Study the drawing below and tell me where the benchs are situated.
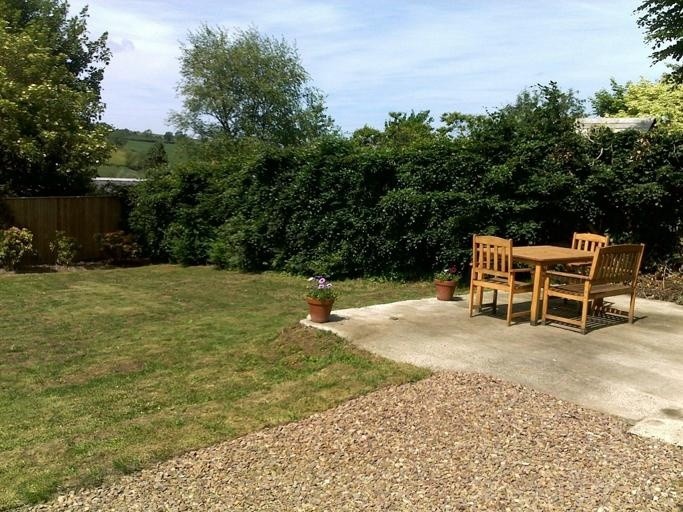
[542,242,644,334]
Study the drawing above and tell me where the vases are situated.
[306,296,333,322]
[434,279,457,301]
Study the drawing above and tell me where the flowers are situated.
[432,265,463,282]
[306,275,338,301]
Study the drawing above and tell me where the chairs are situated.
[469,232,608,326]
